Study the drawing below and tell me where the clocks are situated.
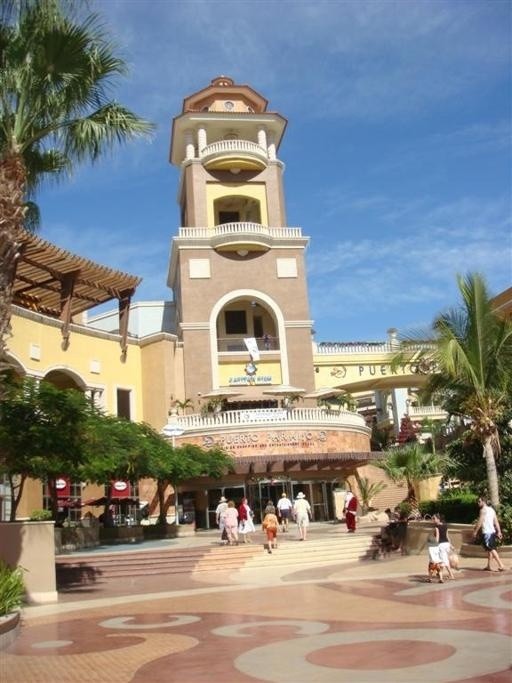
[245,362,256,375]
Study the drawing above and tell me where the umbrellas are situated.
[198,383,346,398]
[57,497,144,527]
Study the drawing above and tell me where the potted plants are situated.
[368,419,467,502]
[437,487,480,523]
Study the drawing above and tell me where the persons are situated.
[342,491,357,533]
[384,509,396,522]
[263,332,272,350]
[215,492,313,553]
[424,535,456,583]
[472,496,507,572]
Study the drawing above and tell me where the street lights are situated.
[161,407,187,524]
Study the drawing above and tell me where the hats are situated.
[296,492,306,498]
[219,496,228,503]
